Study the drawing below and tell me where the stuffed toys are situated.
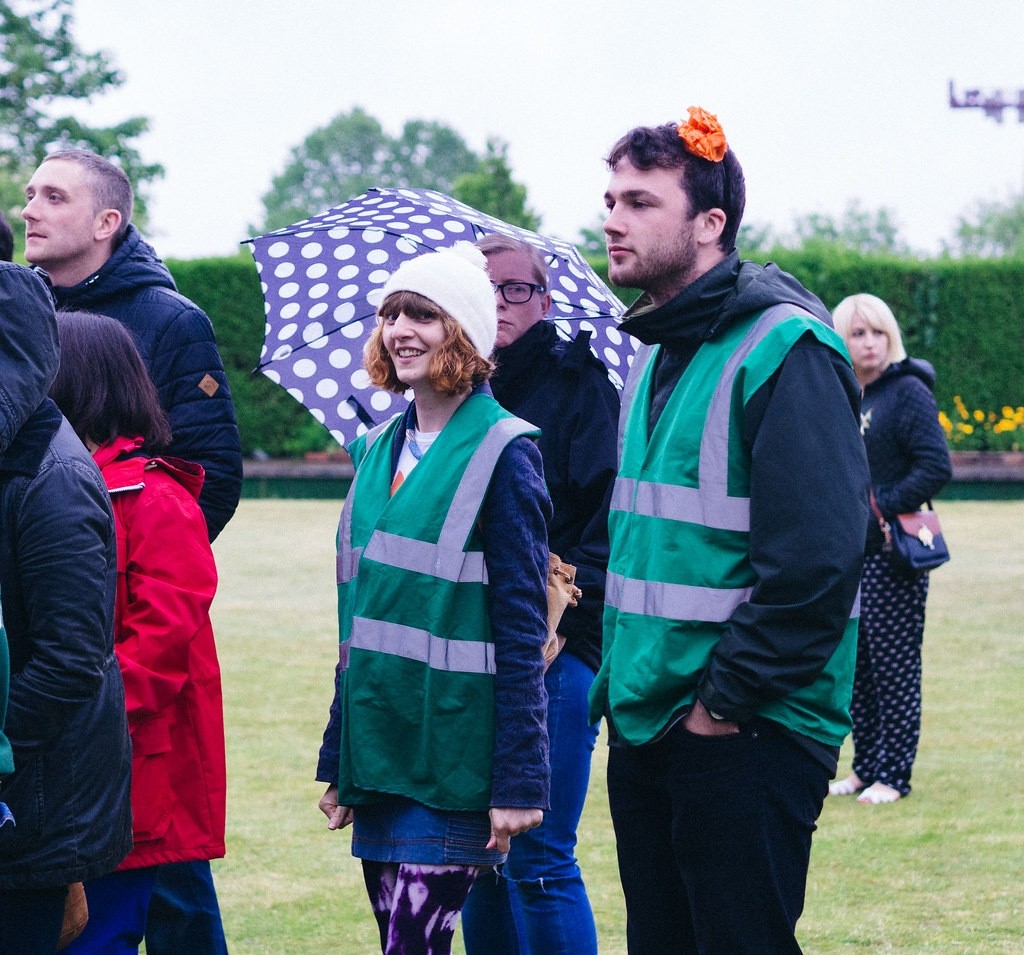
[540,553,582,670]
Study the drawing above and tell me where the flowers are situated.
[676,106,728,162]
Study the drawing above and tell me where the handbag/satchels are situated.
[869,488,950,571]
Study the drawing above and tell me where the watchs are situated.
[709,711,727,721]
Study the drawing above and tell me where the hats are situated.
[380,239,498,359]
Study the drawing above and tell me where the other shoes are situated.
[856,781,902,806]
[829,771,870,796]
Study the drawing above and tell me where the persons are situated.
[461,234,621,955]
[47,312,227,955]
[0,261,136,955]
[588,105,872,955]
[315,241,553,955]
[20,149,243,544]
[828,294,953,805]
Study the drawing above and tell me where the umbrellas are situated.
[240,186,643,454]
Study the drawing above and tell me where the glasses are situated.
[496,281,545,305]
